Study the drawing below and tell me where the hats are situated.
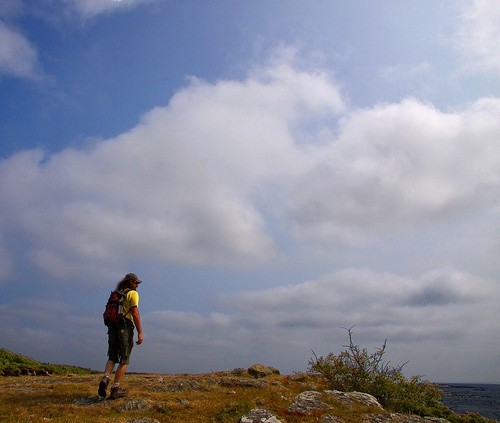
[125,273,142,284]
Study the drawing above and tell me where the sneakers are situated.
[98,377,108,398]
[111,386,128,399]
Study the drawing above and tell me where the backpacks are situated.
[103,290,123,328]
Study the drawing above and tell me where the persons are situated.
[98,273,143,399]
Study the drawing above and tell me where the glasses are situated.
[133,282,138,285]
